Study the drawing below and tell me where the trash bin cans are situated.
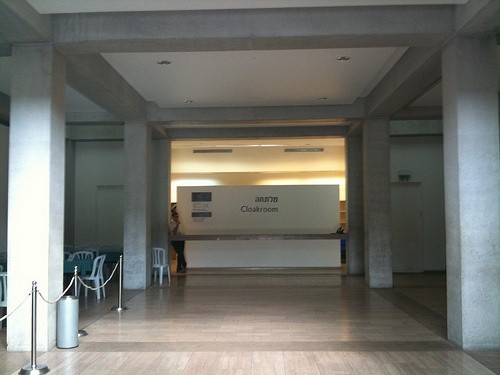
[55,294,80,349]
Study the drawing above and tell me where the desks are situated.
[97,249,124,280]
[63,259,99,297]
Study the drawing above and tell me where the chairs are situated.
[64,242,122,261]
[0,252,8,332]
[151,247,170,286]
[75,254,107,301]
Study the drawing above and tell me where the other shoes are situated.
[176,268,186,273]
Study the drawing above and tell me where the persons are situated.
[168,212,191,273]
